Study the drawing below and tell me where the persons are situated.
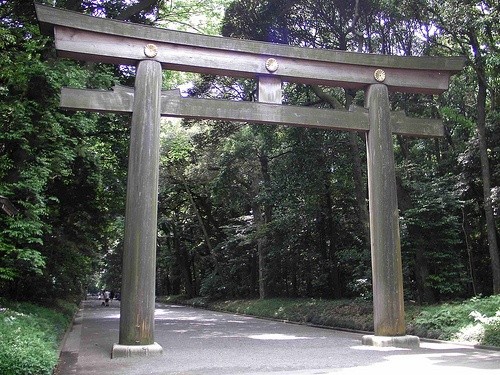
[97,289,120,306]
